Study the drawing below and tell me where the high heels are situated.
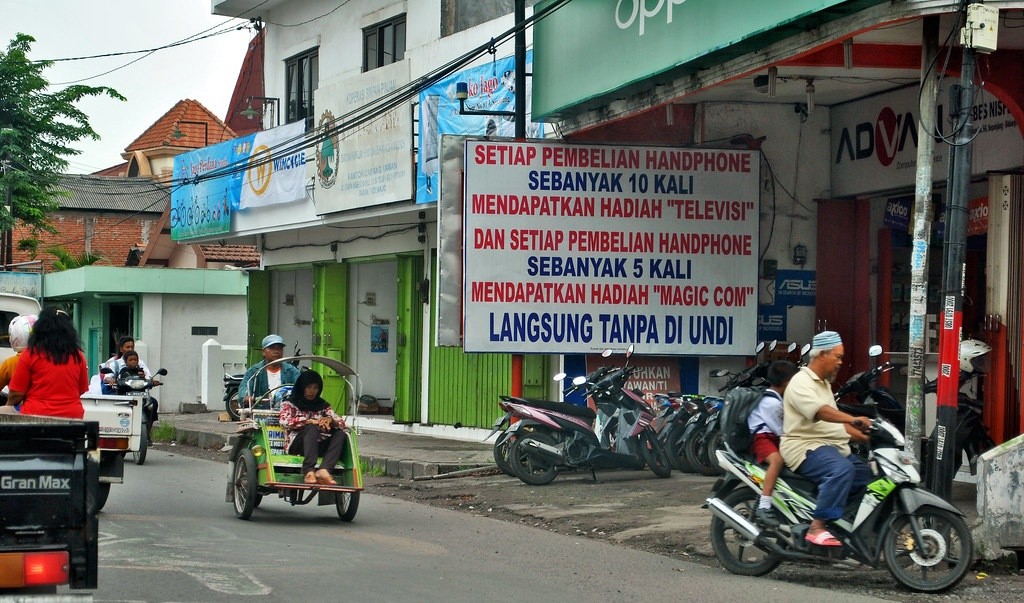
[317,476,338,485]
[304,475,316,483]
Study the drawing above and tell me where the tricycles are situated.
[221,356,363,520]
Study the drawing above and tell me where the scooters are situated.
[222,339,306,421]
[484,343,996,488]
[100,366,169,465]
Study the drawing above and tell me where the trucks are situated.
[0,293,144,512]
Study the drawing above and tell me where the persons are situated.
[109,350,139,386]
[746,360,796,526]
[0,313,40,392]
[279,370,345,486]
[238,335,300,410]
[779,331,873,546]
[100,356,118,395]
[103,337,160,428]
[6,304,89,420]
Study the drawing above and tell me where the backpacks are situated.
[720,387,781,454]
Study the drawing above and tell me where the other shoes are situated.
[148,436,153,446]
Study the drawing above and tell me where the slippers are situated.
[805,531,842,546]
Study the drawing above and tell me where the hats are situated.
[813,331,842,349]
[262,335,286,349]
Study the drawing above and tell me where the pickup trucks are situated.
[0,414,104,603]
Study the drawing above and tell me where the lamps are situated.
[170,120,209,147]
[239,95,280,126]
[455,81,516,119]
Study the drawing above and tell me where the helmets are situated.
[958,339,992,372]
[8,314,39,351]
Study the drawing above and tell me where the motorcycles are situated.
[696,399,975,593]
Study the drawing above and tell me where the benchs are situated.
[250,411,354,481]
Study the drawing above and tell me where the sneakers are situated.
[756,508,779,526]
